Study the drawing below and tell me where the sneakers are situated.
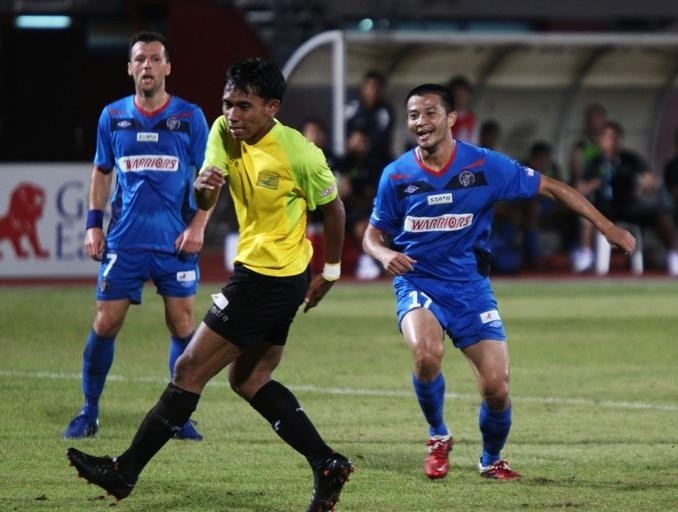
[478,456,525,481]
[63,410,100,441]
[423,432,454,478]
[306,451,356,511]
[172,420,205,441]
[66,446,136,503]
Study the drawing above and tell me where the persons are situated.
[362,82,636,479]
[65,55,355,512]
[65,29,209,440]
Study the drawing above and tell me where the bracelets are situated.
[85,210,105,229]
